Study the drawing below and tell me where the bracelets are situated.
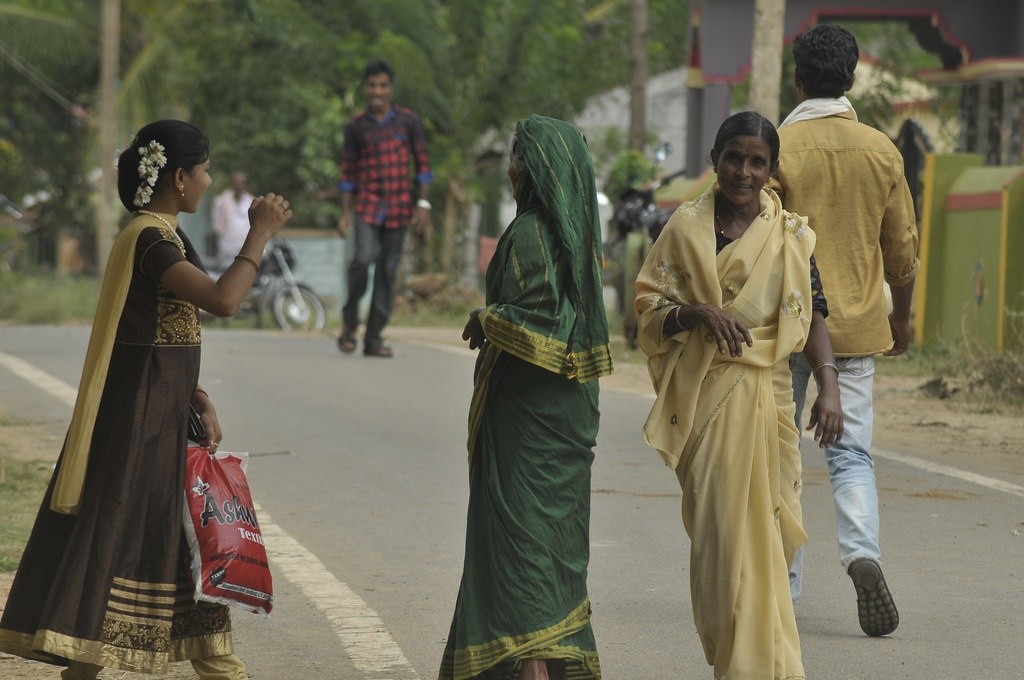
[813,362,840,386]
[196,384,209,397]
[235,254,259,274]
[416,198,432,210]
[675,306,687,332]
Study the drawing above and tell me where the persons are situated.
[0,120,295,680]
[210,173,270,273]
[337,61,432,357]
[605,204,653,348]
[633,112,844,680]
[438,114,614,680]
[763,26,921,635]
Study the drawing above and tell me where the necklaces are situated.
[132,210,186,254]
[715,201,726,246]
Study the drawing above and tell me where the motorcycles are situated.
[591,142,692,247]
[194,227,325,337]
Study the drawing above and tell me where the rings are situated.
[212,442,218,447]
[281,203,286,211]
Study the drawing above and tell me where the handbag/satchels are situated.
[183,439,273,617]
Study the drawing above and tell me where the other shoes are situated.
[848,558,899,636]
[364,340,392,356]
[339,320,356,352]
